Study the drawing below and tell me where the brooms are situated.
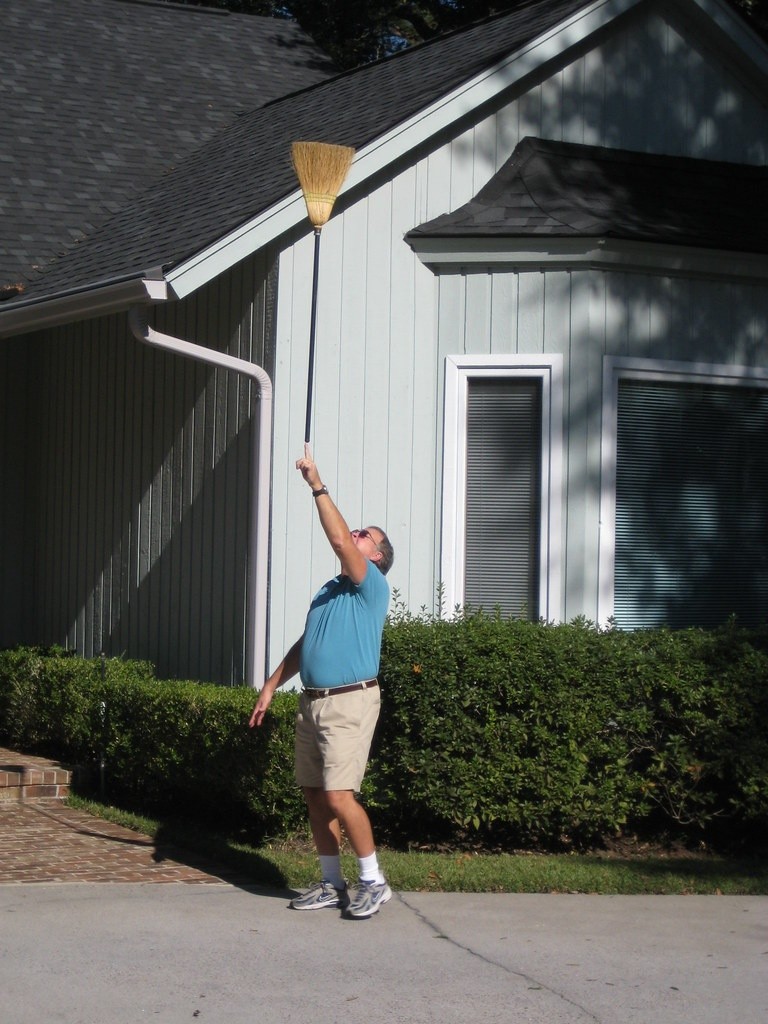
[288,139,357,442]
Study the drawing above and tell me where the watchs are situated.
[313,484,329,497]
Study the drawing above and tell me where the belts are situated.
[307,679,377,698]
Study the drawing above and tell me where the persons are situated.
[246,442,394,920]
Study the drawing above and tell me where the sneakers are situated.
[290,878,351,910]
[345,876,392,918]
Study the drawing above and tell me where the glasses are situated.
[350,529,382,552]
